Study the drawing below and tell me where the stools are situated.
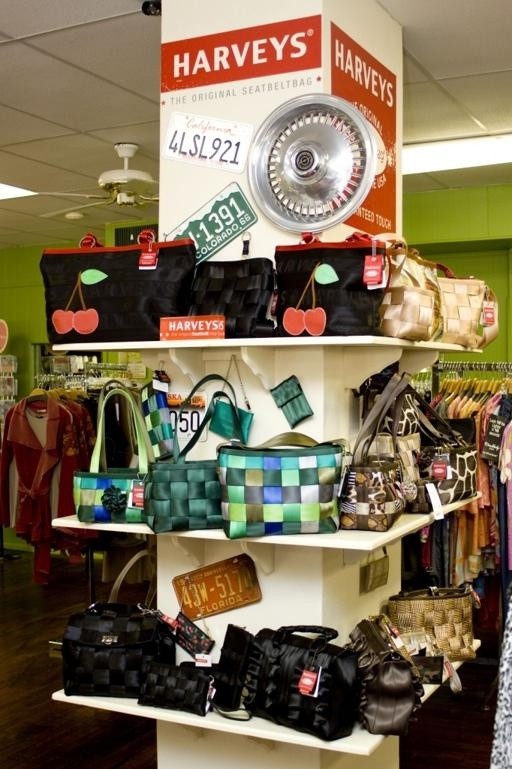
[48,523,136,651]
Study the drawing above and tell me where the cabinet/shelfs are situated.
[51,335,484,769]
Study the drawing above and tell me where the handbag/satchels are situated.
[374,432,422,484]
[39,229,196,344]
[340,453,407,532]
[208,399,253,444]
[187,257,275,338]
[71,464,156,525]
[365,556,390,593]
[273,231,391,337]
[360,385,420,435]
[376,240,445,343]
[436,263,500,351]
[210,623,267,721]
[217,434,354,540]
[344,614,425,736]
[170,575,215,662]
[144,458,246,535]
[389,583,482,661]
[137,661,217,717]
[270,375,313,430]
[419,419,477,447]
[253,626,362,742]
[138,370,176,461]
[410,656,444,684]
[405,442,482,515]
[61,602,175,696]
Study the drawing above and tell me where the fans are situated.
[38,142,159,218]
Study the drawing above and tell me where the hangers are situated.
[25,373,89,402]
[87,362,128,382]
[437,361,512,405]
